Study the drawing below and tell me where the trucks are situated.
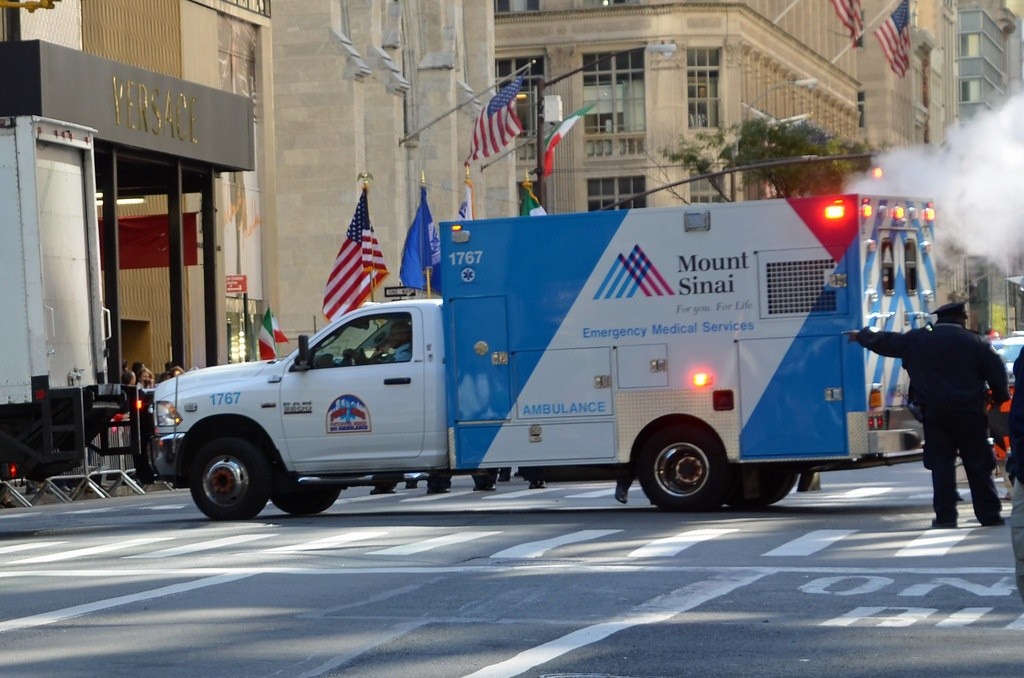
[1,116,143,511]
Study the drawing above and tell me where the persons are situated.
[615,477,638,505]
[841,299,1010,529]
[375,322,412,364]
[1006,344,1024,609]
[120,360,200,487]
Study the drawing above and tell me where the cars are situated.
[984,330,1024,415]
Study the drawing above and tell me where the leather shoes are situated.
[614,484,628,503]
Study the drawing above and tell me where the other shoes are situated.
[981,516,1005,526]
[932,518,959,528]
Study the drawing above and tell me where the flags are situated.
[457,179,476,221]
[520,186,548,216]
[833,0,866,48]
[399,186,442,296]
[323,186,390,322]
[543,103,600,178]
[465,67,524,167]
[873,0,910,78]
[259,306,288,360]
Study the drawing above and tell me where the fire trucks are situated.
[146,192,949,521]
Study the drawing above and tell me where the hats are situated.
[930,300,968,319]
